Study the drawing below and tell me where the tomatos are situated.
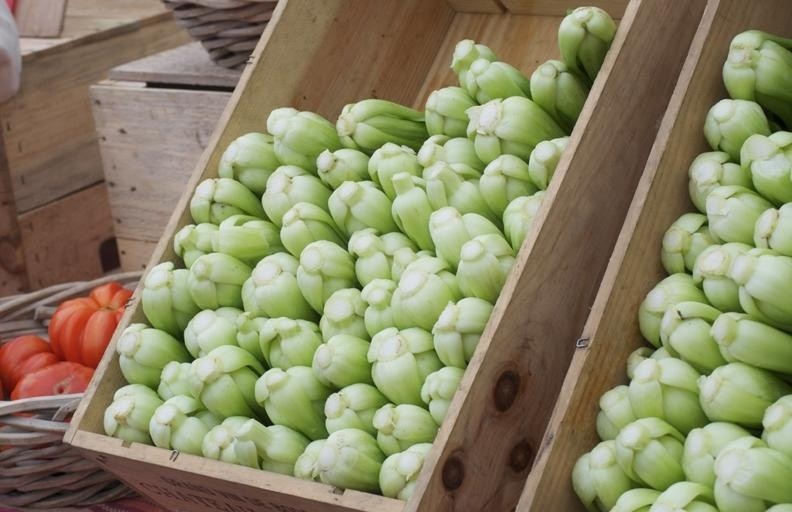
[0,280,139,453]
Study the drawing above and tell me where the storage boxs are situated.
[63,0,705,512]
[2,0,195,298]
[84,39,259,271]
[515,1,792,511]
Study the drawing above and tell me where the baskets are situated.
[2,271,142,512]
[160,0,277,72]
